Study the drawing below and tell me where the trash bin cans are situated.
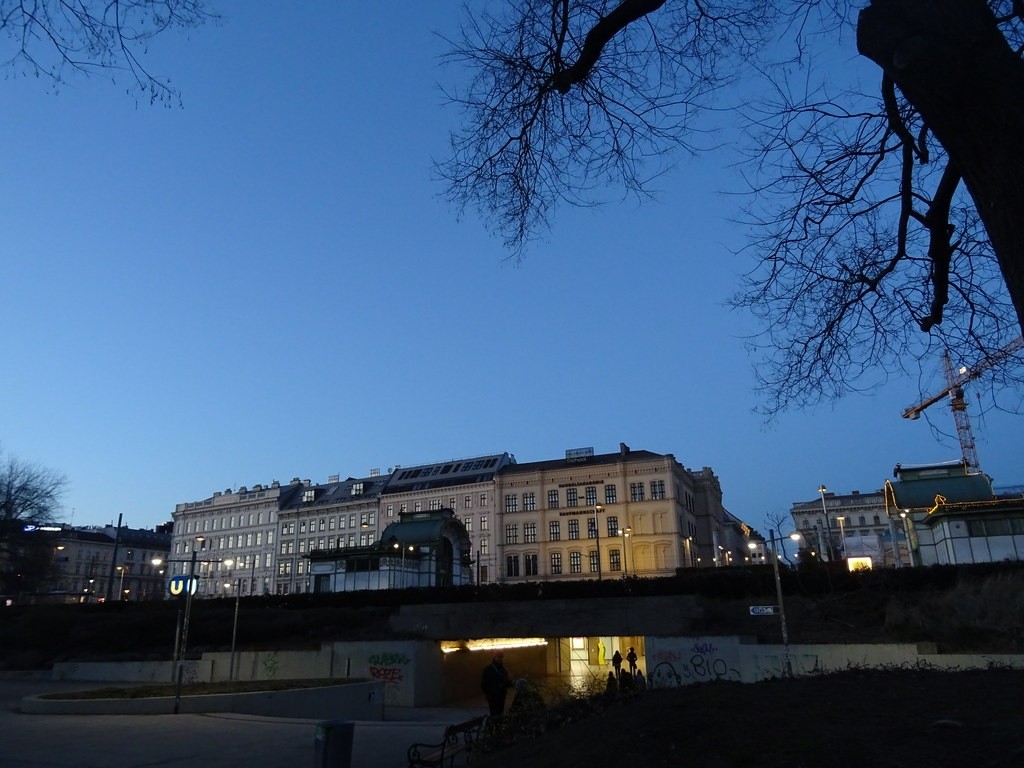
[314,721,357,768]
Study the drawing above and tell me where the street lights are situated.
[148,549,237,716]
[836,514,848,559]
[594,501,603,581]
[239,559,256,595]
[223,578,243,683]
[747,530,801,701]
[817,484,836,560]
[618,525,633,575]
[393,541,415,589]
[116,565,125,600]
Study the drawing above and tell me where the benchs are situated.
[407,714,488,768]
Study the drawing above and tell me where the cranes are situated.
[898,334,1024,474]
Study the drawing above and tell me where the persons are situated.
[509,678,544,735]
[480,650,517,735]
[603,669,646,695]
[626,647,637,679]
[612,651,623,676]
